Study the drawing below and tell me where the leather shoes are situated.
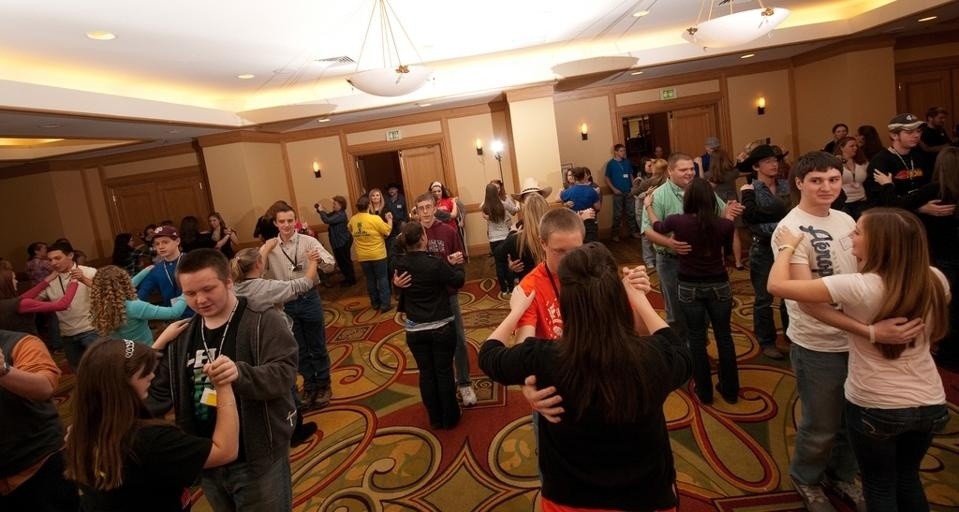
[693,385,714,404]
[430,405,463,430]
[734,261,745,270]
[290,422,317,448]
[761,346,784,358]
[715,383,738,405]
[372,303,394,312]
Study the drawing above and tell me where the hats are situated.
[511,178,553,203]
[738,144,789,173]
[705,137,720,149]
[888,113,928,132]
[151,226,179,238]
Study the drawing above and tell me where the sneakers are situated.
[459,385,478,408]
[612,235,620,242]
[339,277,357,288]
[646,263,655,269]
[632,231,641,238]
[790,477,866,512]
[311,384,332,409]
[299,388,317,409]
[502,287,510,296]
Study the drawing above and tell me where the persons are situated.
[641,152,747,404]
[389,193,477,431]
[604,137,745,269]
[346,181,470,313]
[729,105,958,509]
[315,196,357,288]
[477,207,692,509]
[1,200,334,511]
[480,166,601,300]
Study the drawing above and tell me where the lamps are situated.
[312,161,321,178]
[681,0,792,50]
[581,122,588,140]
[757,96,766,115]
[476,139,483,155]
[491,139,504,186]
[346,0,432,97]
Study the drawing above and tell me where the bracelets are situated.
[869,324,876,344]
